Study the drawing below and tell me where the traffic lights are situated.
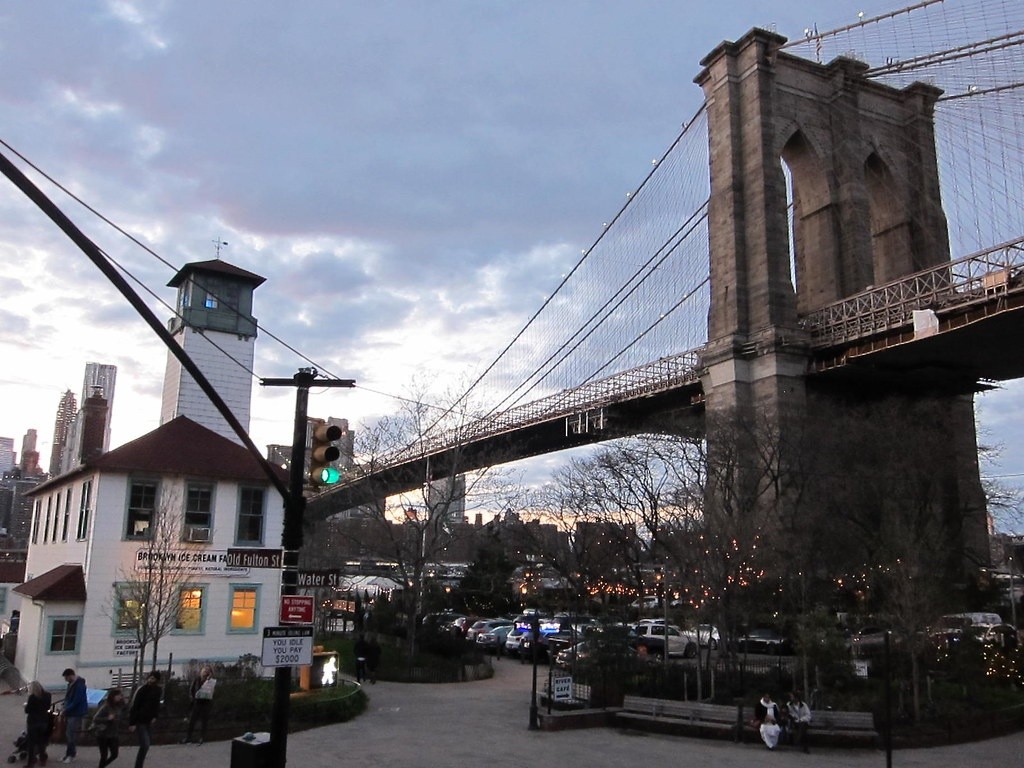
[309,422,342,487]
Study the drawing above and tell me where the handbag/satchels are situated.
[195,678,217,700]
[749,719,762,729]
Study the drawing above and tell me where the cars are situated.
[628,595,666,608]
[719,627,791,653]
[420,608,667,669]
[629,623,700,659]
[680,623,732,651]
[844,624,905,648]
[911,613,1019,655]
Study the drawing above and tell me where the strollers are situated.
[7,707,61,763]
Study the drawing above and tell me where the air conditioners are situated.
[186,526,211,543]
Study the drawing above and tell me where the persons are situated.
[786,692,812,754]
[129,672,162,768]
[755,693,782,751]
[354,633,367,683]
[22,681,51,768]
[367,634,380,684]
[93,690,128,768]
[182,667,215,744]
[56,669,88,763]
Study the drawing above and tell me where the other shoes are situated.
[803,748,810,754]
[177,736,192,745]
[57,753,79,764]
[197,738,203,746]
[768,747,777,751]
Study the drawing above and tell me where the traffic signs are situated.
[278,595,315,625]
[554,677,573,701]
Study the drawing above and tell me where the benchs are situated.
[110,670,172,688]
[616,694,739,741]
[533,681,591,707]
[740,706,878,748]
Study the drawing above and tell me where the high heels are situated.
[23,756,38,768]
[40,754,48,767]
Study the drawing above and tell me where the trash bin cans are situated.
[231,732,270,768]
